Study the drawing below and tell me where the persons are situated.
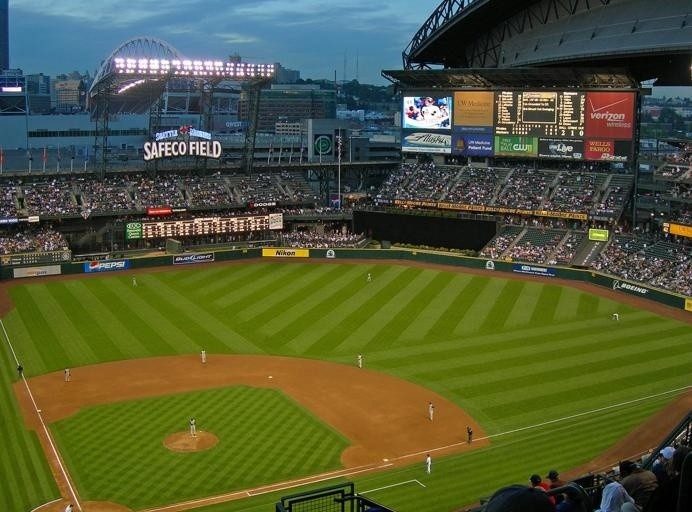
[65,504,73,512]
[407,97,449,126]
[480,422,691,512]
[189,417,196,438]
[358,354,362,368]
[425,453,432,473]
[428,402,434,421]
[17,365,23,375]
[200,348,206,363]
[466,426,472,444]
[64,367,70,382]
[612,313,618,320]
[2,144,692,297]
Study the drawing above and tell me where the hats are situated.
[660,447,676,459]
[471,484,554,512]
